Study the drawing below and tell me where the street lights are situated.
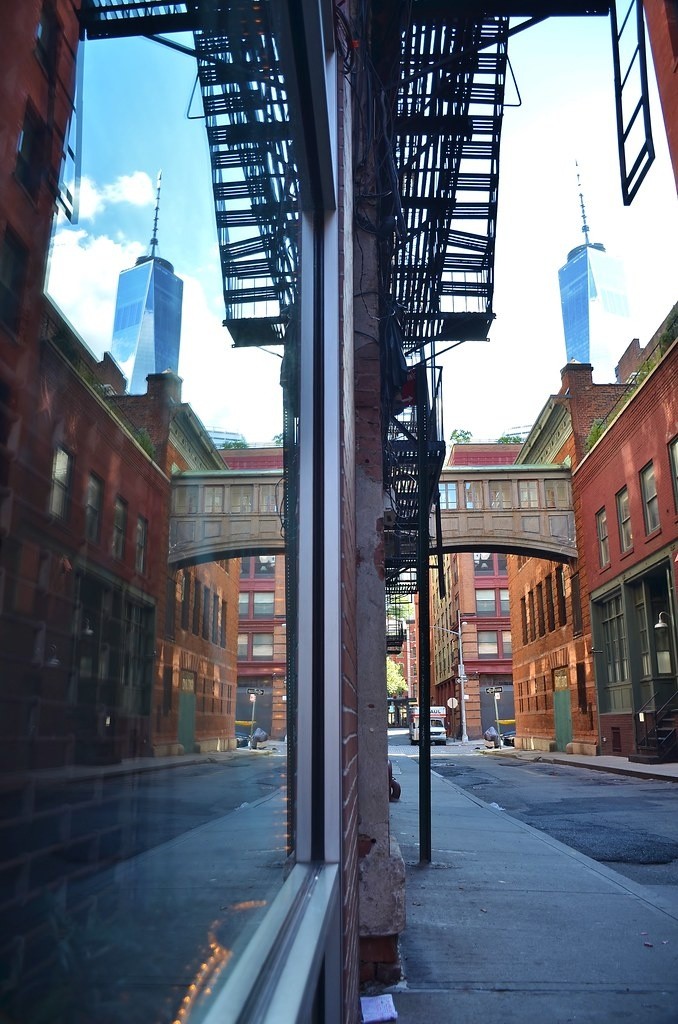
[416,608,470,744]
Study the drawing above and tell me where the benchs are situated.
[76,732,125,767]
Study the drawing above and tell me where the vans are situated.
[406,704,448,743]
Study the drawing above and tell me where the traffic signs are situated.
[486,687,503,693]
[246,688,264,695]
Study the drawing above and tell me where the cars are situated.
[500,730,516,747]
[235,731,250,749]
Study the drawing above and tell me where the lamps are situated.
[654,612,671,629]
[81,616,94,636]
[46,643,61,668]
